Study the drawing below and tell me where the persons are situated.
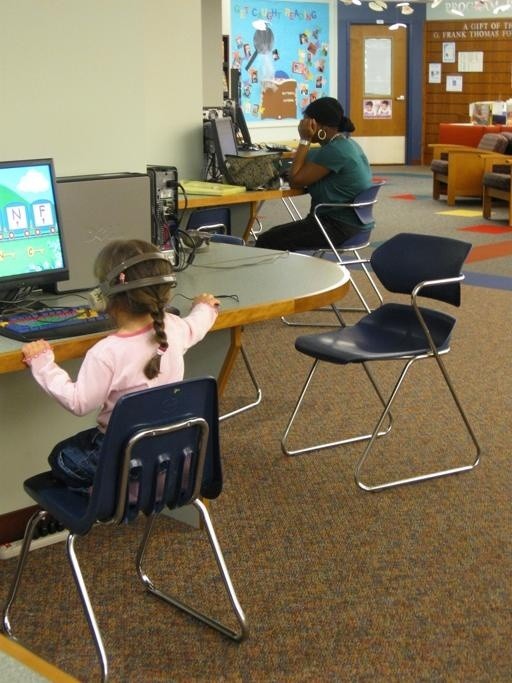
[364,101,376,117]
[254,96,374,251]
[472,103,495,123]
[19,239,221,510]
[378,100,391,117]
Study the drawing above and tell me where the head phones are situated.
[88,252,177,313]
[180,229,210,254]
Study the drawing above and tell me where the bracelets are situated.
[299,139,310,146]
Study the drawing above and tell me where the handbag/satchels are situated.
[223,150,284,191]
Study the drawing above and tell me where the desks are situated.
[0,241,353,425]
[172,180,310,244]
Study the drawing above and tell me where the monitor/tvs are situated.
[1,158,69,314]
[237,107,260,150]
[211,116,238,176]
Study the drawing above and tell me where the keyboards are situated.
[0,301,179,342]
[265,143,292,151]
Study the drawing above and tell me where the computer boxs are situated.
[203,106,225,181]
[147,162,177,251]
[42,173,150,296]
[225,99,239,135]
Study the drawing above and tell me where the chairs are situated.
[278,231,482,494]
[278,178,386,329]
[427,133,511,221]
[3,375,250,683]
